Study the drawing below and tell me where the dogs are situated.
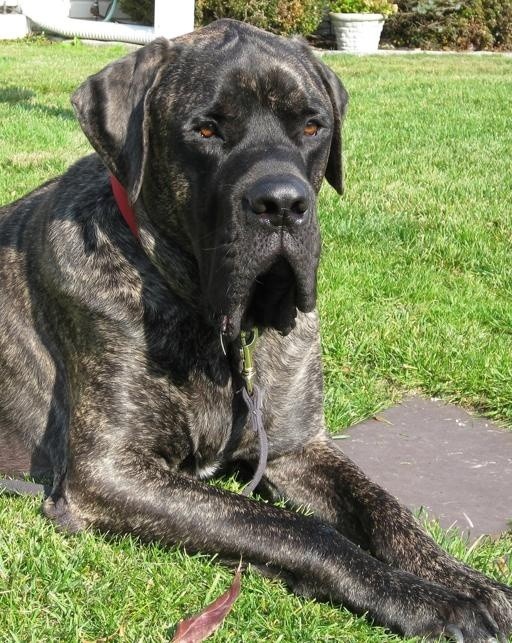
[0,18,512,643]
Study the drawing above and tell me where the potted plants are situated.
[328,0,397,55]
